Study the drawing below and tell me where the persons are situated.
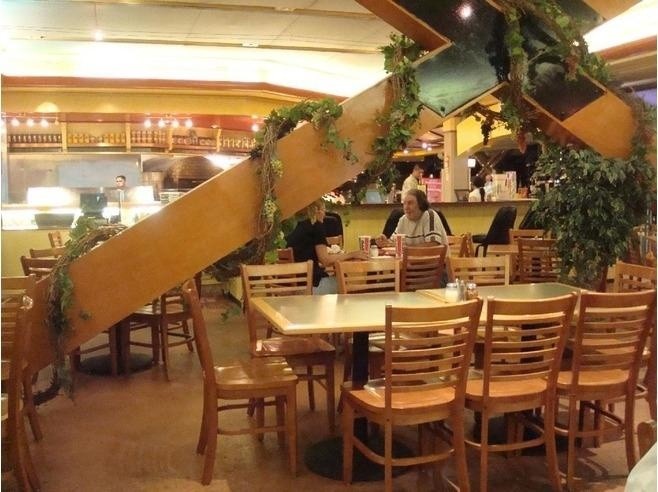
[284,197,369,294]
[110,176,130,202]
[375,189,449,288]
[468,176,487,203]
[400,164,424,203]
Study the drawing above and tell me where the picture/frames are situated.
[453,188,471,202]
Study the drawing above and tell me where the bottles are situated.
[132,130,215,146]
[445,278,465,303]
[370,244,379,257]
[466,282,479,300]
[67,132,126,145]
[7,135,62,142]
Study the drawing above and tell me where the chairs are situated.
[560,259,657,452]
[178,275,301,486]
[1,273,47,492]
[508,286,657,491]
[19,254,111,370]
[221,199,562,445]
[424,290,580,492]
[337,294,486,492]
[30,246,66,258]
[118,280,194,383]
[46,231,65,247]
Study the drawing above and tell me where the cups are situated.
[395,233,406,258]
[358,236,371,256]
[220,135,255,150]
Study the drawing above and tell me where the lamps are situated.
[2,112,59,128]
[142,114,195,131]
